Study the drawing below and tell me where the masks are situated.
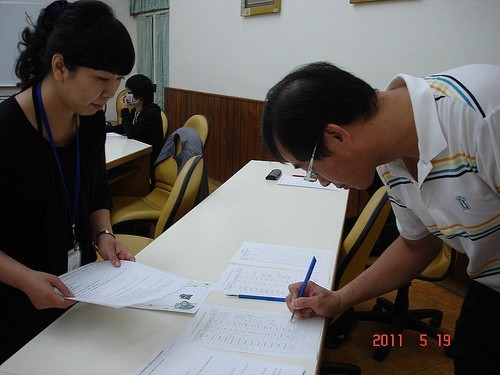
[125,94,142,106]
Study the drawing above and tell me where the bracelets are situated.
[92,230,117,251]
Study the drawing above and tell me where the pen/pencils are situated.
[292,174,306,178]
[291,255,318,320]
[225,294,286,303]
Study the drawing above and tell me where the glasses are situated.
[127,90,133,94]
[304,146,318,182]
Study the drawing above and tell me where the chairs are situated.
[321,186,458,375]
[96,89,209,263]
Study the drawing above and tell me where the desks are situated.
[105,132,153,169]
[0,160,349,375]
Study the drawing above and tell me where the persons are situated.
[104,74,163,197]
[260,62,500,375]
[0,0,135,365]
